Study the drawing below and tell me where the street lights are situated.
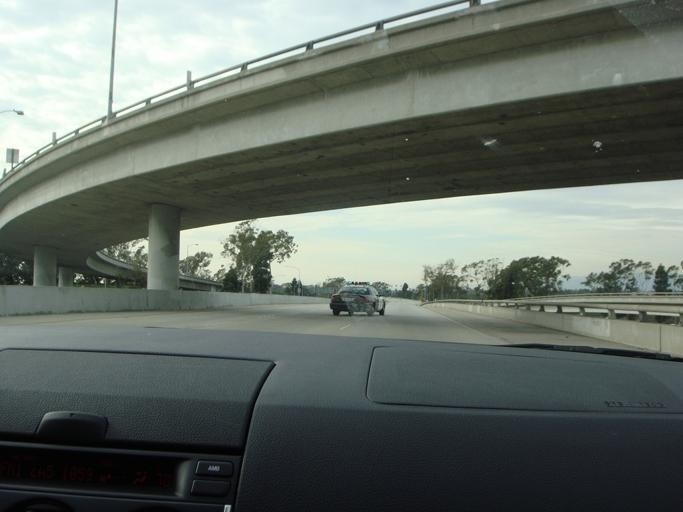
[0,108,23,116]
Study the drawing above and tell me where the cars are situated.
[330,283,385,317]
